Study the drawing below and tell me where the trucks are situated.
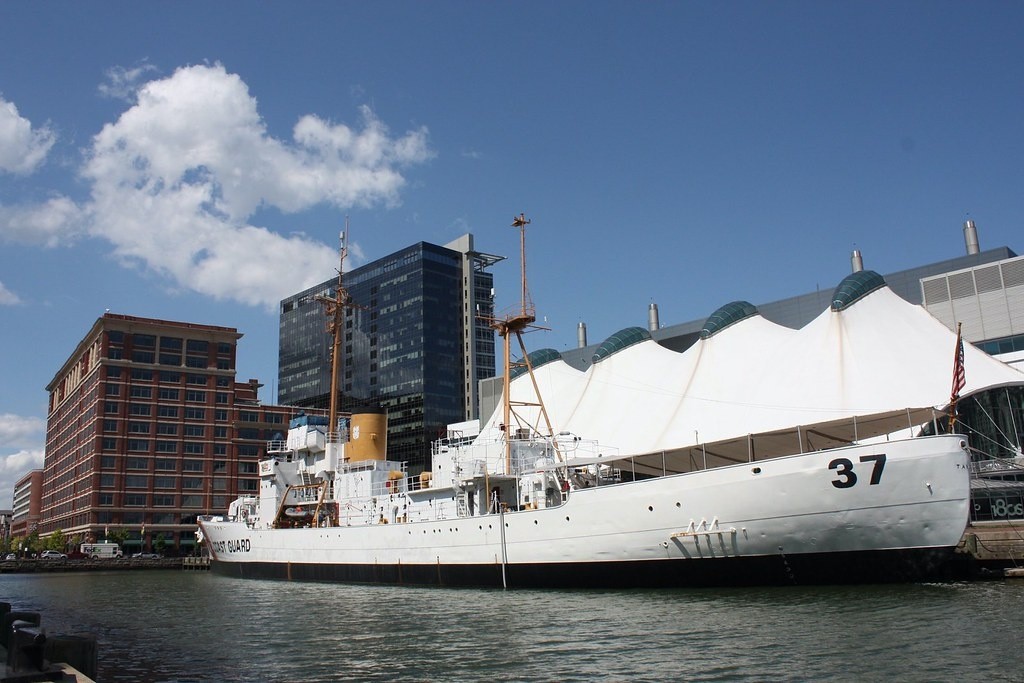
[80,543,123,559]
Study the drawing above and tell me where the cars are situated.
[132,552,162,559]
[6,553,16,560]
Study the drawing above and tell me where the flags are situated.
[951,329,966,401]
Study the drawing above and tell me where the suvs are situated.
[41,550,68,560]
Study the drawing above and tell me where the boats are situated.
[195,214,971,565]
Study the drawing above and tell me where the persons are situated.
[291,504,336,529]
